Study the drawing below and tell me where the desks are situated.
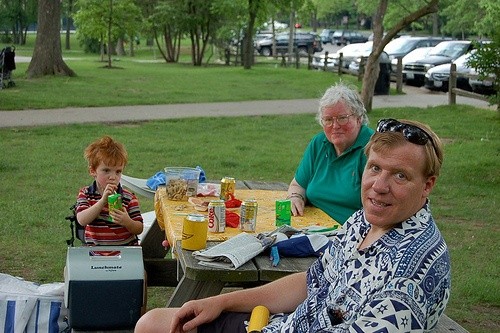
[139,181,342,308]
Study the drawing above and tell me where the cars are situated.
[315,37,500,91]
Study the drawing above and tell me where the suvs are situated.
[332,30,368,46]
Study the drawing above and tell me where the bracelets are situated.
[286,191,304,201]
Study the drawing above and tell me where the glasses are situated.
[376,118,439,157]
[320,113,354,126]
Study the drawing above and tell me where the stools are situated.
[432,312,470,333]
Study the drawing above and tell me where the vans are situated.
[257,31,322,56]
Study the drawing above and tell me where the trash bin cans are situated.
[375,51,393,94]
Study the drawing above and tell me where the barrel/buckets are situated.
[165,167,201,202]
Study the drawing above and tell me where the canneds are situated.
[220,176,235,200]
[240,200,258,231]
[208,199,225,233]
[186,213,206,221]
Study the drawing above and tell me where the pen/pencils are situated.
[174,213,205,217]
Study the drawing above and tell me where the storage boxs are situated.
[64,246,145,328]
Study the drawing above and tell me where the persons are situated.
[285,78,375,224]
[134,117,452,332]
[75,135,144,245]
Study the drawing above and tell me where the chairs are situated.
[66,205,140,246]
